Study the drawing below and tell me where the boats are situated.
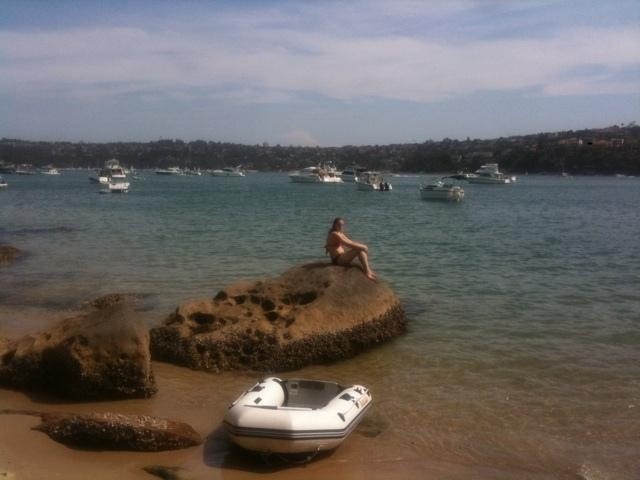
[0,162,61,191]
[419,176,464,202]
[439,161,516,184]
[289,157,391,191]
[153,164,248,176]
[224,377,372,457]
[98,157,135,192]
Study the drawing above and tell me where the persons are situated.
[324,217,379,283]
